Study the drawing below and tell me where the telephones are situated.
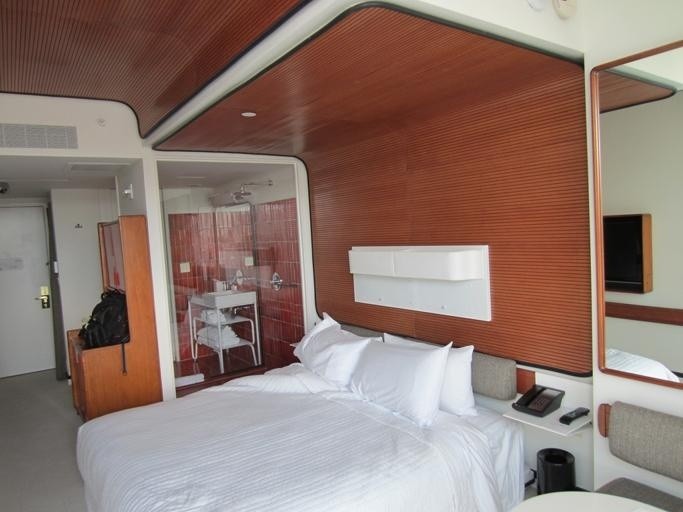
[512,384,565,417]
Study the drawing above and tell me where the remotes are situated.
[559,407,590,425]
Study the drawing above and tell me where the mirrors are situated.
[589,41,683,392]
[214,202,258,292]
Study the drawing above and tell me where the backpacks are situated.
[78,285,130,349]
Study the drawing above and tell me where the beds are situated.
[74,325,525,512]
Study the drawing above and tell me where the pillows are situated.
[291,311,479,426]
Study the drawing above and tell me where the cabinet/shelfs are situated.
[187,301,261,376]
[66,214,163,422]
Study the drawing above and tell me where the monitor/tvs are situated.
[602,214,652,293]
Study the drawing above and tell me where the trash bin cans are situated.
[537,448,575,494]
